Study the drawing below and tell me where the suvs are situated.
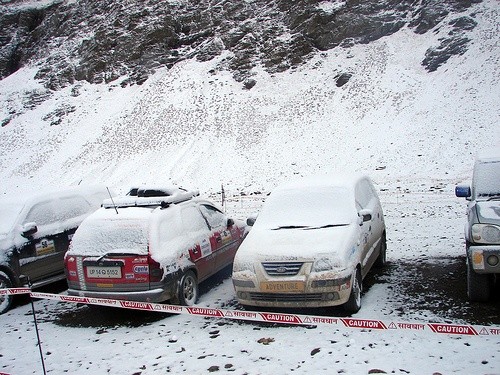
[455,148,500,301]
[63,184,250,307]
[0,186,104,315]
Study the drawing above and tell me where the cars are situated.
[231,172,388,314]
